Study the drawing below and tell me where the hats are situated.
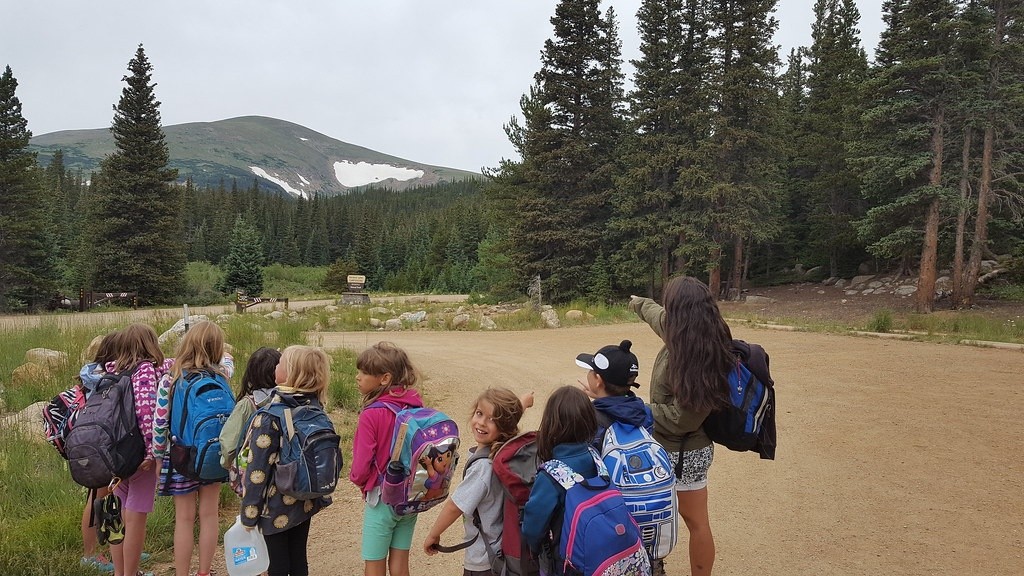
[575,340,640,389]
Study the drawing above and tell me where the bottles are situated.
[386,461,412,484]
[171,436,179,446]
[223,514,270,576]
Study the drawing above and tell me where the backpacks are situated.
[466,431,558,576]
[170,366,239,484]
[258,390,343,500]
[536,445,653,576]
[43,380,90,460]
[703,339,772,453]
[593,405,677,560]
[63,358,150,489]
[364,401,461,517]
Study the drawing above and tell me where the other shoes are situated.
[136,570,154,576]
[94,493,125,545]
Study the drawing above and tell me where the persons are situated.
[219,348,281,470]
[72,321,235,576]
[628,275,734,576]
[241,345,331,576]
[349,341,423,576]
[424,388,534,576]
[522,385,599,576]
[576,340,665,576]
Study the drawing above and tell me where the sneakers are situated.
[80,554,115,572]
[141,552,150,559]
